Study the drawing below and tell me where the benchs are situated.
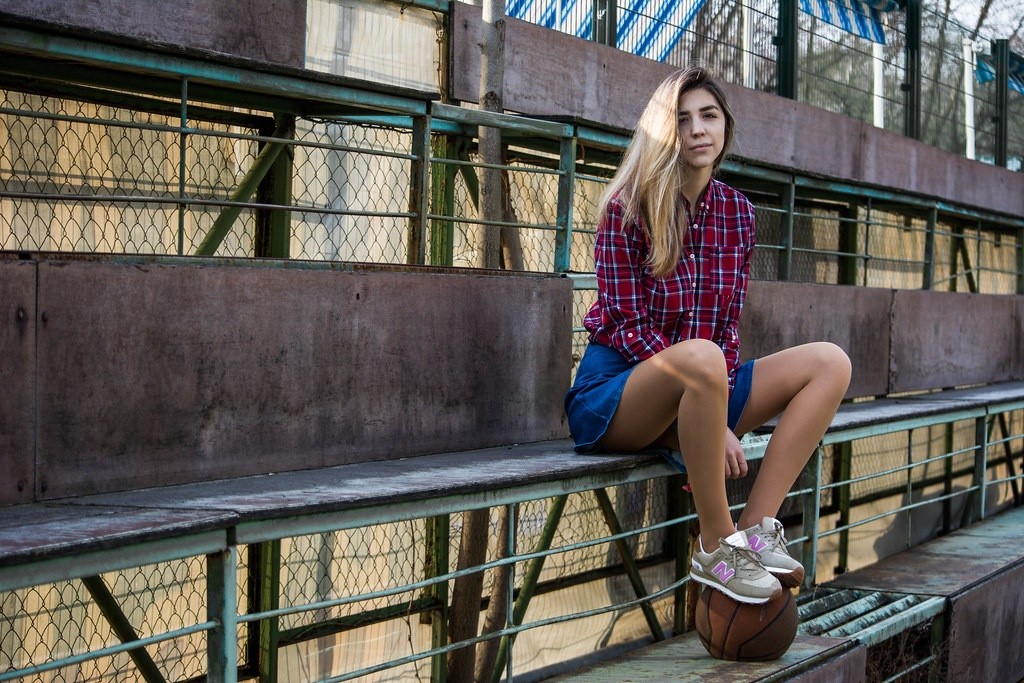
[0,250,1024,683]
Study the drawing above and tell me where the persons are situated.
[564,67,852,604]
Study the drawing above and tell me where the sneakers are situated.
[689,530,783,605]
[735,517,805,589]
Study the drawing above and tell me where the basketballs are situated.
[693,581,799,663]
